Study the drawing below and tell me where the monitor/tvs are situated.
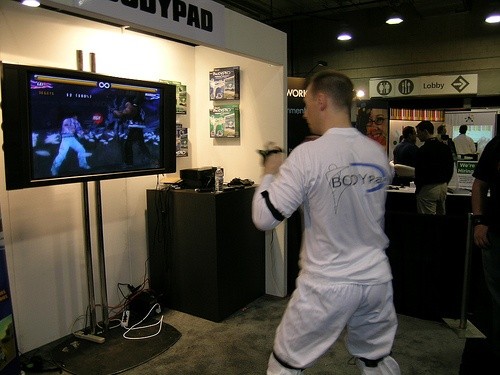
[0,63,177,191]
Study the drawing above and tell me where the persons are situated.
[393,126,420,189]
[252,70,399,375]
[436,124,448,144]
[471,133,500,289]
[452,124,476,155]
[414,121,455,215]
[367,108,390,150]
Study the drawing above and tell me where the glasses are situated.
[367,117,388,126]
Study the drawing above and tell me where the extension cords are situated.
[74,331,105,343]
[121,310,130,327]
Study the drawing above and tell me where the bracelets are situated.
[472,208,483,215]
